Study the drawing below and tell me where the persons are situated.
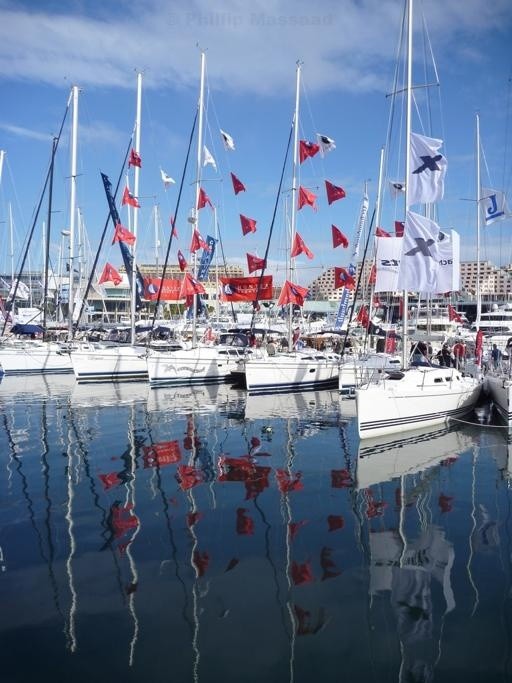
[490,344,503,369]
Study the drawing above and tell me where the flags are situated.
[490,189,505,227]
[94,132,483,367]
